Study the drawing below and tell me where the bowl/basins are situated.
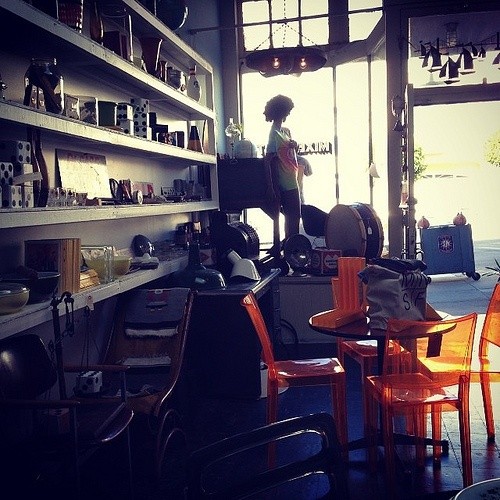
[0,283,30,315]
[0,272,61,303]
[84,259,130,281]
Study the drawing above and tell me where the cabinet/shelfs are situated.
[0,0,222,402]
[193,267,281,401]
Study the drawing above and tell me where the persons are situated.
[264,95,301,237]
[297,155,312,202]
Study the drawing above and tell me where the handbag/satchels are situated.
[357,265,428,332]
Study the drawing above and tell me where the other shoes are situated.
[285,271,307,277]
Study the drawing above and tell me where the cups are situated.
[113,255,131,275]
[48,185,88,206]
[236,139,252,158]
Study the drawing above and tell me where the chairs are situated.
[0,257,500,500]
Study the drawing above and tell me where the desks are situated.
[309,309,457,450]
[217,157,283,247]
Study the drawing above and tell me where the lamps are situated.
[445,22,458,48]
[245,0,329,76]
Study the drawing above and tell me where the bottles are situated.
[187,124,202,152]
[225,117,239,159]
[27,0,187,95]
[186,60,200,102]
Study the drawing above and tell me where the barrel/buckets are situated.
[98,101,117,127]
